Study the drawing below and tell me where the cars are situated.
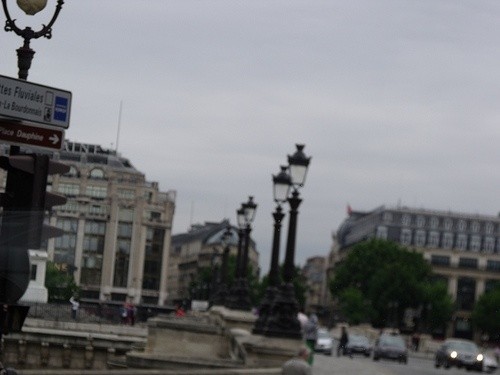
[373,335,409,363]
[433,339,483,372]
[313,331,334,356]
[343,334,373,357]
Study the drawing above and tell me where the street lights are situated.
[262,143,313,338]
[228,195,258,311]
[216,228,232,305]
[250,165,293,335]
[224,204,246,308]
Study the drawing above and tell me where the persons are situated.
[303,309,318,365]
[412,329,421,352]
[481,333,500,361]
[119,303,139,326]
[70,296,80,319]
[176,304,185,318]
[336,326,349,357]
